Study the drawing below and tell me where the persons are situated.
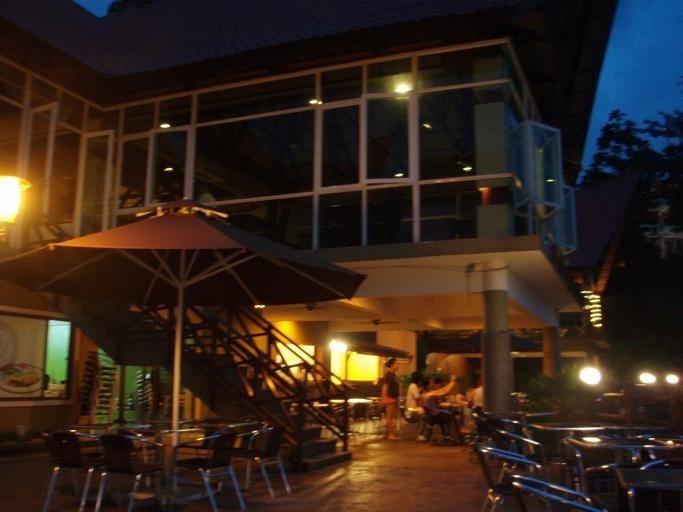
[380,356,401,440]
[403,371,485,443]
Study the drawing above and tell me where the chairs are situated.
[40,416,292,510]
[474,395,683,511]
[291,395,485,448]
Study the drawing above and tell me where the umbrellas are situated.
[0,195,367,490]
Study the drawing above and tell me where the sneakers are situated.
[417,434,461,445]
[385,432,399,440]
